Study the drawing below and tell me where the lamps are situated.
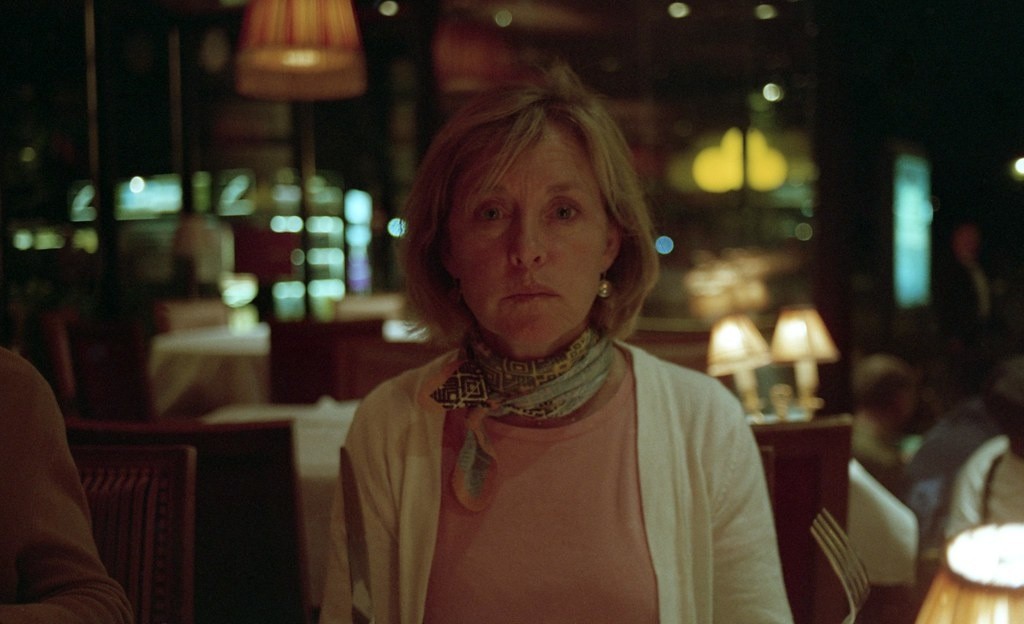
[706,304,841,422]
[235,0,368,103]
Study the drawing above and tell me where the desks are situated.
[145,322,270,415]
[205,401,358,607]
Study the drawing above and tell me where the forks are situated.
[811,508,872,624]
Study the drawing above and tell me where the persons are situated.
[936,222,996,355]
[318,65,793,624]
[849,353,917,489]
[0,346,135,624]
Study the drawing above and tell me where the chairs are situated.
[75,445,197,624]
[63,413,321,624]
